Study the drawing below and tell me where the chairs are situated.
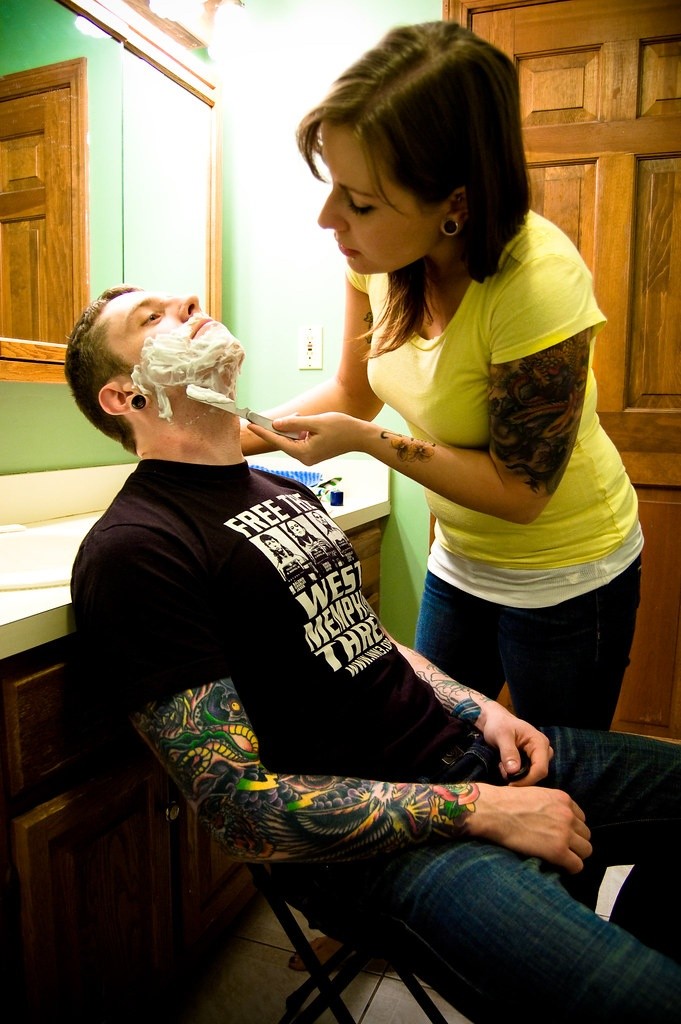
[245,860,446,1022]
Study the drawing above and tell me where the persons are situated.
[240,20,645,972]
[64,285,681,1024]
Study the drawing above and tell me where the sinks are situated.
[1,534,82,580]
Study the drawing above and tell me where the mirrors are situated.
[0,0,228,387]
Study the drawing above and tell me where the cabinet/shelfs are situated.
[0,631,181,1023]
[180,733,259,964]
[345,525,381,619]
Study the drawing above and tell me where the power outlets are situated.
[297,324,323,370]
